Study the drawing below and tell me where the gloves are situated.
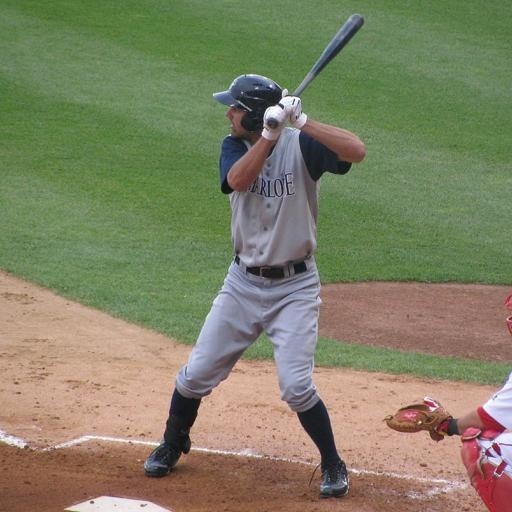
[260,107,286,142]
[278,96,308,131]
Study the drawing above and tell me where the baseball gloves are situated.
[385,397,454,439]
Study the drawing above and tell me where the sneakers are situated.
[316,458,347,498]
[143,441,181,478]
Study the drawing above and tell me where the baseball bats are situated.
[268,13,364,130]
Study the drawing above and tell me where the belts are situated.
[233,252,307,278]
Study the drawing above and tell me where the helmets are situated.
[209,72,286,114]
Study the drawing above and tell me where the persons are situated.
[383,287,512,512]
[144,74,367,497]
